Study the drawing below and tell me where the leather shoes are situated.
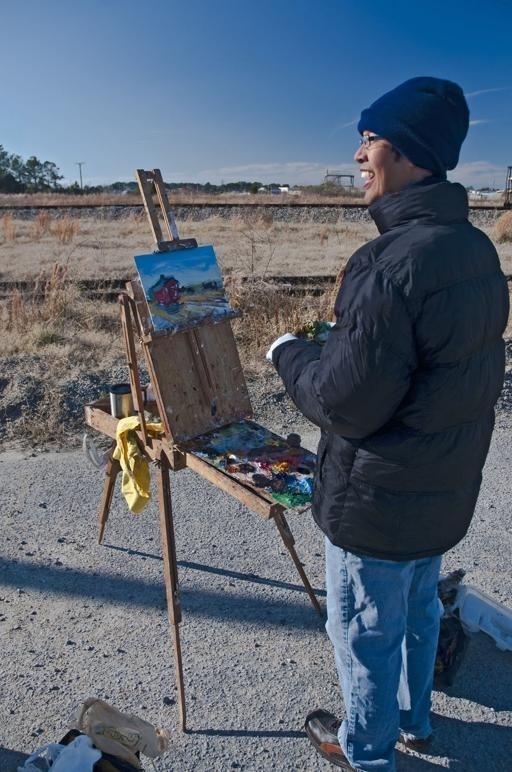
[305,707,357,772]
[396,733,433,753]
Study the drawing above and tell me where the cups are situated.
[109,382,148,419]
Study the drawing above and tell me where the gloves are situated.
[315,322,337,348]
[265,330,299,365]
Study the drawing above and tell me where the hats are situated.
[357,75,470,175]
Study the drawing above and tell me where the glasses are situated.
[358,134,389,149]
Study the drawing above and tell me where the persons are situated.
[266,76,510,772]
